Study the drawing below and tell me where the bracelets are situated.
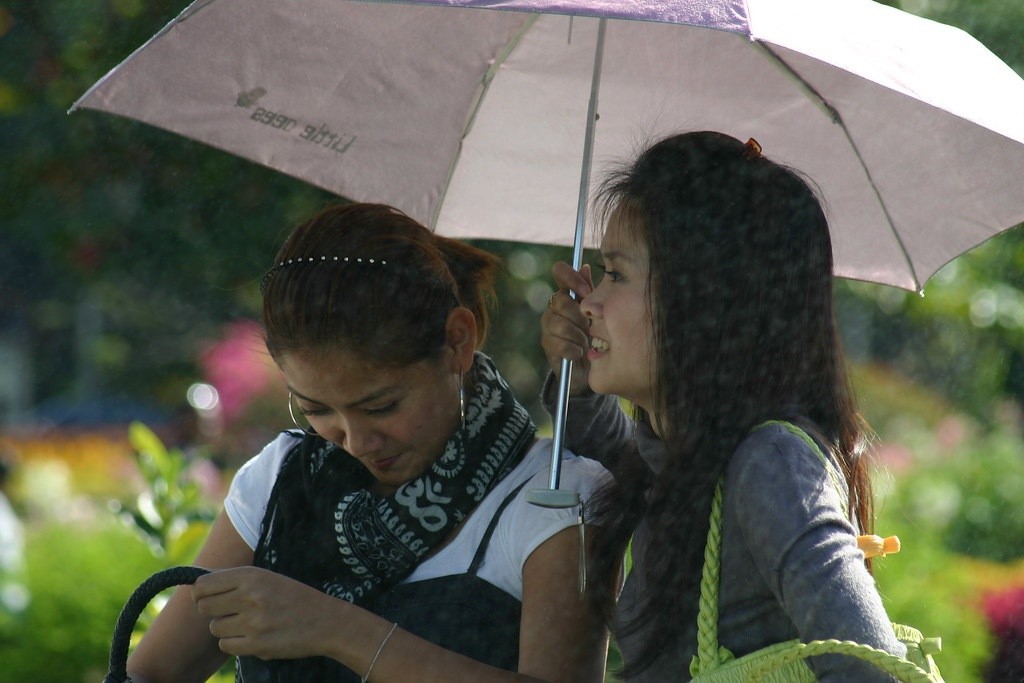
[362,623,398,683]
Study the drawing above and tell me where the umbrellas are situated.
[67,0,1024,508]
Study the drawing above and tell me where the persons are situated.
[539,131,908,683]
[127,203,626,683]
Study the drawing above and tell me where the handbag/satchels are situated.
[689,421,947,683]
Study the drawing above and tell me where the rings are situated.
[549,293,558,308]
